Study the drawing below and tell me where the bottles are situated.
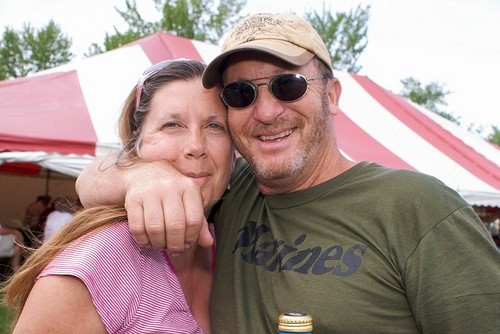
[278,312,313,334]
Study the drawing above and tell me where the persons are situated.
[75,13,500,334]
[0,193,81,272]
[0,57,238,334]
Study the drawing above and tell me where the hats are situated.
[201,12,333,90]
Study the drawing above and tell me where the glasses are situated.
[219,71,332,110]
[134,58,200,112]
